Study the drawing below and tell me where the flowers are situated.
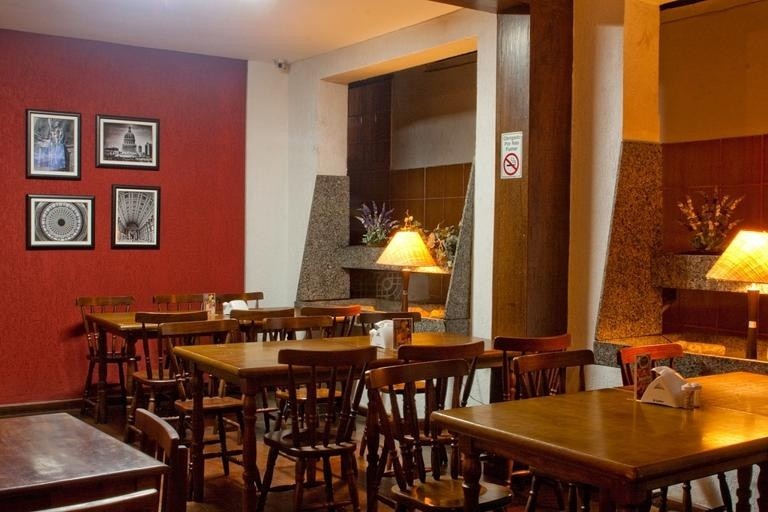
[350,199,398,247]
[673,181,743,254]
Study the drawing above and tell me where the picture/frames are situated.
[25,108,82,182]
[109,182,161,250]
[95,113,160,172]
[24,194,96,251]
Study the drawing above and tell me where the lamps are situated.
[374,228,438,309]
[704,228,767,360]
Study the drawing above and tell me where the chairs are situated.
[395,340,487,499]
[616,343,735,512]
[492,334,573,401]
[506,348,596,511]
[364,352,516,511]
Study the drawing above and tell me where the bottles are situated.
[223,302,231,314]
[680,382,702,409]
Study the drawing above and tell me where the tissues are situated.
[223,298,249,314]
[640,365,687,407]
[371,319,393,347]
